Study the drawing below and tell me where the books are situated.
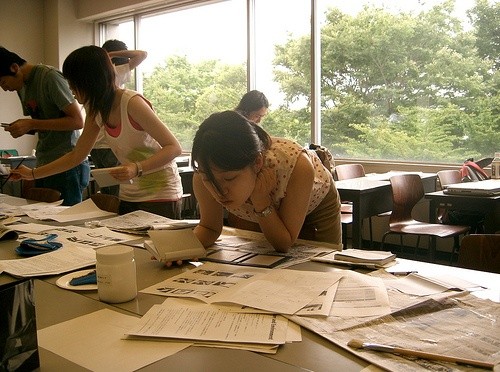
[334,249,396,266]
[91,166,133,188]
[143,229,206,264]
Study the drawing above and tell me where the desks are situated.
[0,156,36,197]
[424,189,500,264]
[177,166,198,219]
[332,171,438,263]
[0,194,500,372]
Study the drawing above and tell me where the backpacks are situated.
[458,158,496,183]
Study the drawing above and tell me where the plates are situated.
[56,269,99,290]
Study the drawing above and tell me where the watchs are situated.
[253,202,274,217]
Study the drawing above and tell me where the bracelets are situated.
[31,167,37,180]
[136,162,143,177]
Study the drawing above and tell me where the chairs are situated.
[335,163,365,180]
[381,175,468,265]
[460,234,500,273]
[20,188,61,203]
[0,149,22,195]
[416,170,463,262]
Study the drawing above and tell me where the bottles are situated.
[96,246,138,303]
[491,152,500,179]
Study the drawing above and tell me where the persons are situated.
[9,45,182,219]
[151,109,343,268]
[81,39,147,212]
[227,90,269,233]
[0,45,89,206]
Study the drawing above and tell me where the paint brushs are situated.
[346,338,495,369]
